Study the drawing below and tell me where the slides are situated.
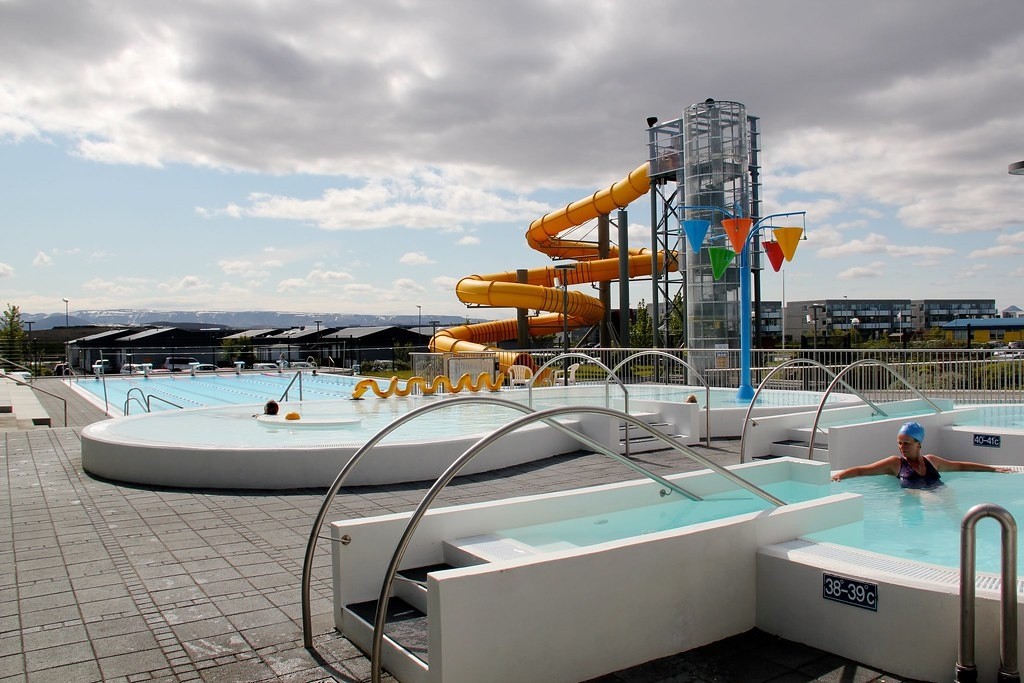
[428,154,681,383]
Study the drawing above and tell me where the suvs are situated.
[53,363,71,376]
[95,359,113,374]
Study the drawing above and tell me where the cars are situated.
[985,339,1024,360]
[190,364,221,370]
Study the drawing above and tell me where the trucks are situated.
[120,364,170,373]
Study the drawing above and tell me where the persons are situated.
[311,369,321,376]
[830,417,1019,530]
[680,392,701,406]
[216,400,279,420]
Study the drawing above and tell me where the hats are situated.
[899,421,925,442]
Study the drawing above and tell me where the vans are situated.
[165,357,201,371]
